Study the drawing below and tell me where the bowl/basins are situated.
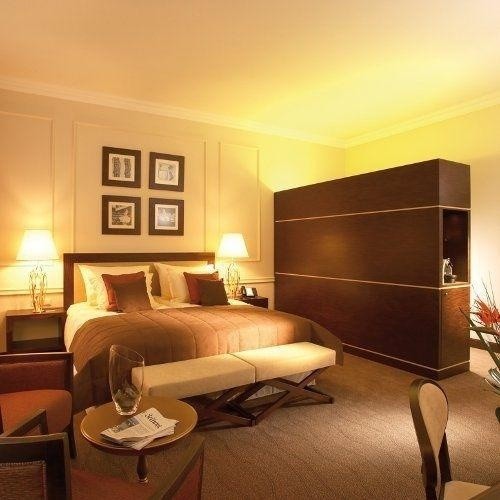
[445,275,457,283]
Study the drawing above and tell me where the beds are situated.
[63,252,344,428]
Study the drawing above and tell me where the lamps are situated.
[216,233,249,293]
[16,229,59,312]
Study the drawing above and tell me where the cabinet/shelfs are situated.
[274,158,471,381]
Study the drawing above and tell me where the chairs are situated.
[1,408,71,500]
[1,352,78,460]
[410,377,492,499]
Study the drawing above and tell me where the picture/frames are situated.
[102,194,141,235]
[149,151,185,193]
[101,146,142,188]
[149,197,184,235]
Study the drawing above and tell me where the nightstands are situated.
[6,310,67,355]
[234,295,268,309]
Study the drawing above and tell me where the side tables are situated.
[80,395,198,485]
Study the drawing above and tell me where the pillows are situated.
[78,262,159,312]
[154,262,229,306]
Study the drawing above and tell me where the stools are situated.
[131,341,336,427]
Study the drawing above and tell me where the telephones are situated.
[241,286,258,298]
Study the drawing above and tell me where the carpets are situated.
[0,408,205,500]
